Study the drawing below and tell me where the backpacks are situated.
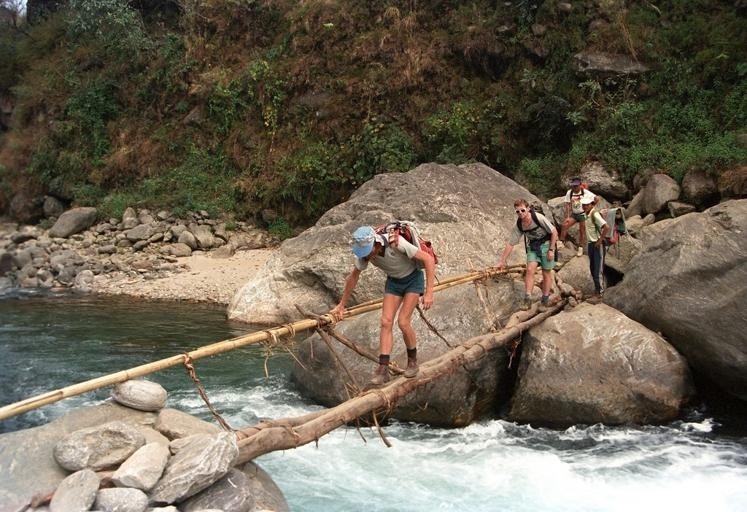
[373,219,439,265]
[591,206,626,245]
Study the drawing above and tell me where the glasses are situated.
[515,208,526,213]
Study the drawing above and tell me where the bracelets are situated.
[549,249,554,252]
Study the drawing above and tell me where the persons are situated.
[498,197,557,311]
[557,178,600,259]
[330,224,434,388]
[579,194,610,297]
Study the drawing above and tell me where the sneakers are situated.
[577,246,584,257]
[371,366,391,385]
[404,358,420,378]
[541,295,549,305]
[558,240,565,249]
[520,299,532,311]
[587,294,602,305]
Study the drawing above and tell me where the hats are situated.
[580,193,594,205]
[570,180,580,185]
[351,225,374,258]
